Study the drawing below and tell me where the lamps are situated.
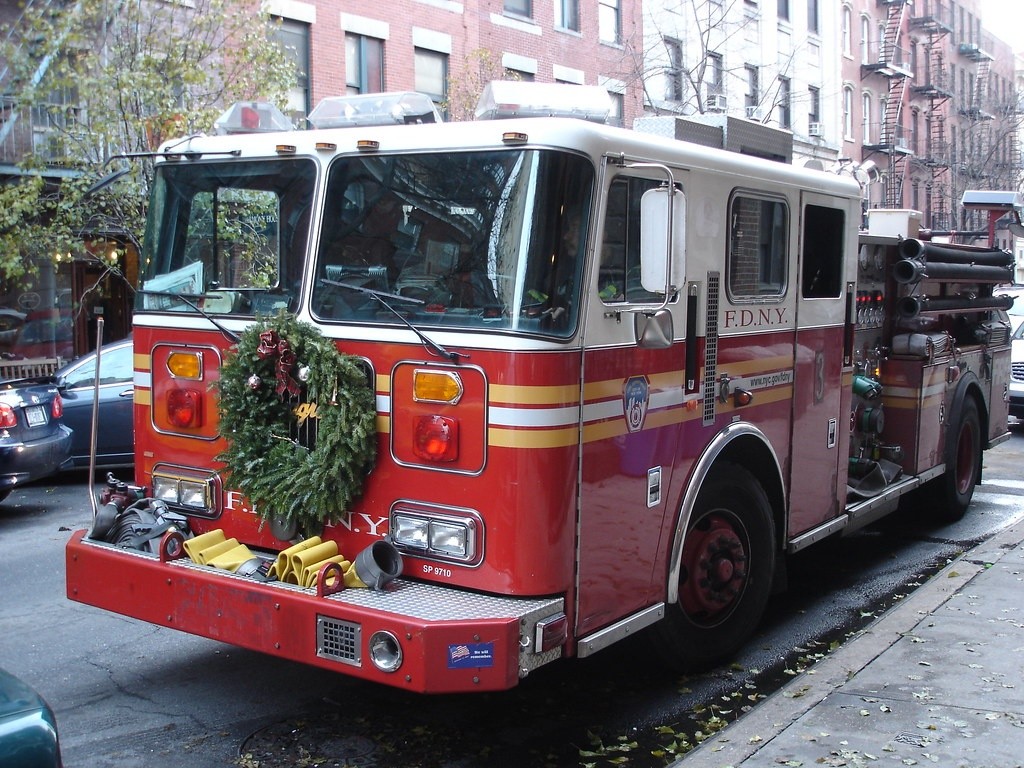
[825,158,881,234]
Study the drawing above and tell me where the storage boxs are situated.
[866,209,923,240]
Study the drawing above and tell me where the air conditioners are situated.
[746,105,763,120]
[809,122,825,136]
[707,93,727,111]
[895,137,907,148]
[897,62,911,70]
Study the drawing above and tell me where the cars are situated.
[44,337,133,478]
[0,371,75,507]
[991,287,1024,435]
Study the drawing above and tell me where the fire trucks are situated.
[60,77,1015,697]
